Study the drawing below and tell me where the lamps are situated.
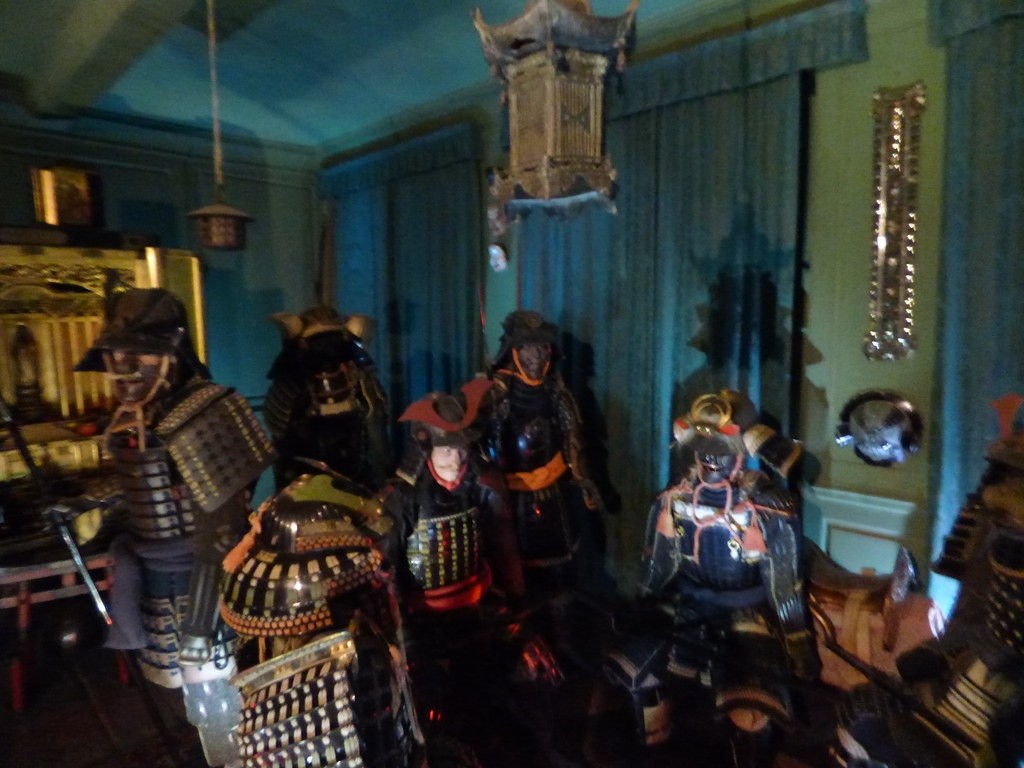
[185,1,258,252]
[471,1,637,215]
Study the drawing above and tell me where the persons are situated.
[20,287,280,768]
[264,305,391,491]
[897,425,1024,768]
[633,386,823,768]
[474,310,602,647]
[219,476,429,768]
[373,376,567,737]
